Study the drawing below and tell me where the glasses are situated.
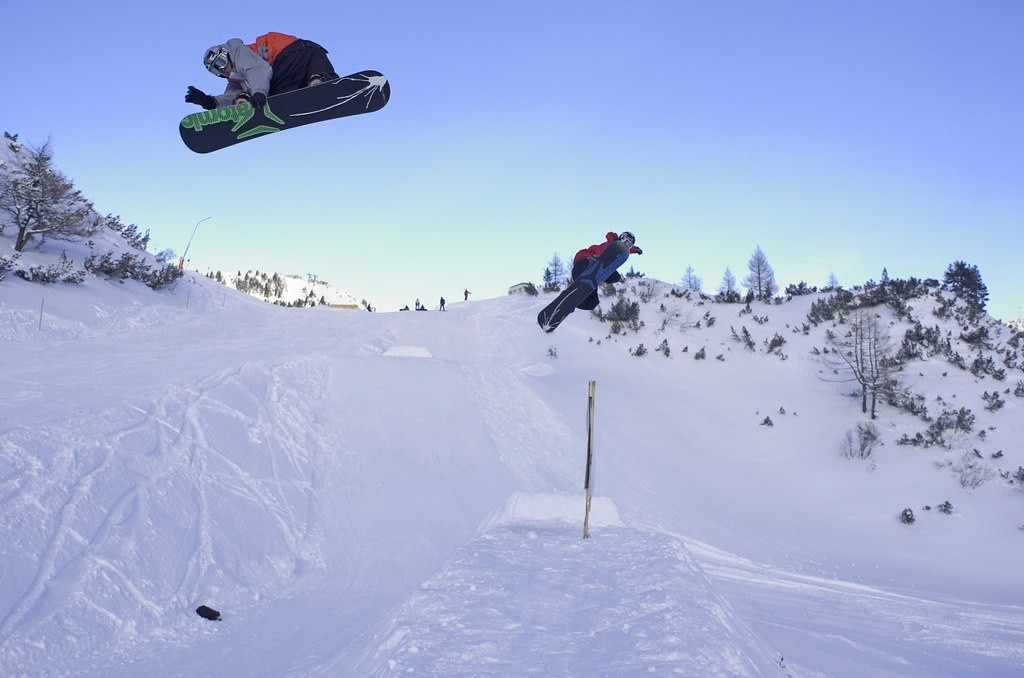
[209,48,228,76]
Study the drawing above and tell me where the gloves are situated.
[636,247,642,255]
[185,86,219,110]
[249,92,266,108]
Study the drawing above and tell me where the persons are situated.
[571,231,644,310]
[185,32,338,111]
[464,289,470,300]
[415,298,420,310]
[440,296,446,312]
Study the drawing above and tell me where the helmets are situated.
[204,45,233,78]
[619,232,635,249]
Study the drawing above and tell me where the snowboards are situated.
[537,240,630,333]
[178,69,392,156]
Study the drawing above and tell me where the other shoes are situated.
[235,91,250,105]
[309,73,326,87]
